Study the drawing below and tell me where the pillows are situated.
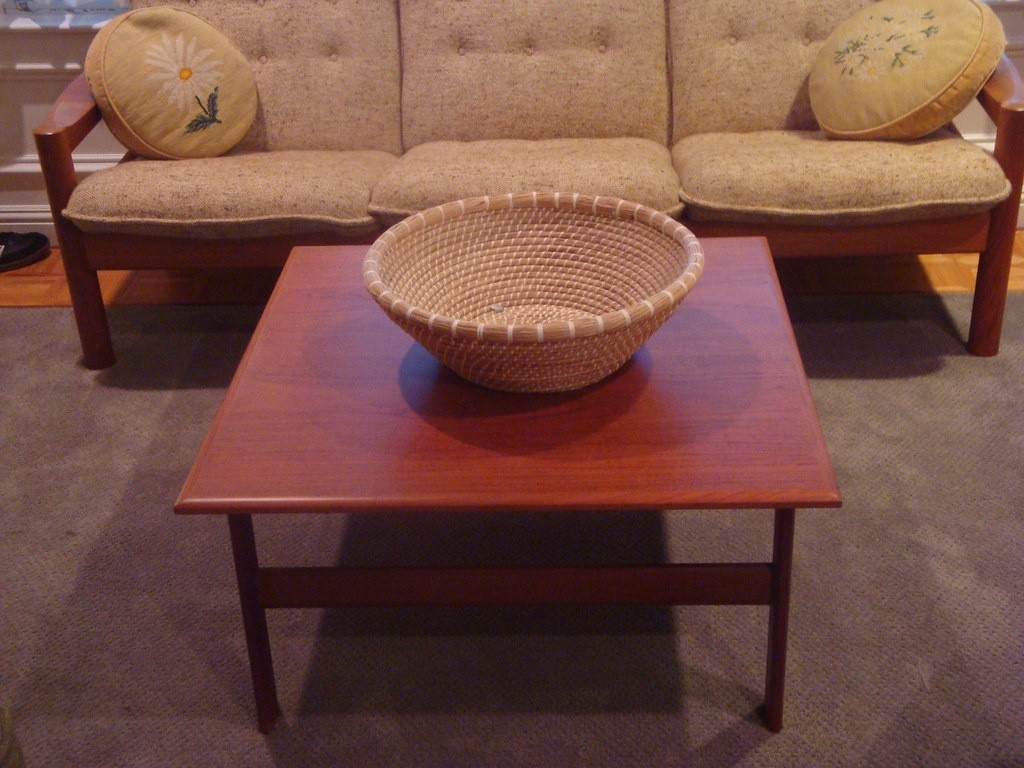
[84,6,258,159]
[809,0,1007,142]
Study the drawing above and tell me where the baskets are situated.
[361,191,704,392]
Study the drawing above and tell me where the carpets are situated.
[1,289,1024,768]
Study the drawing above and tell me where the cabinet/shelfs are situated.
[0,0,127,247]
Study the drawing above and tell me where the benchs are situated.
[34,0,1024,370]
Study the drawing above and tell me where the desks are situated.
[174,234,843,732]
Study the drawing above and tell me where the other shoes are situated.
[0,231,50,273]
